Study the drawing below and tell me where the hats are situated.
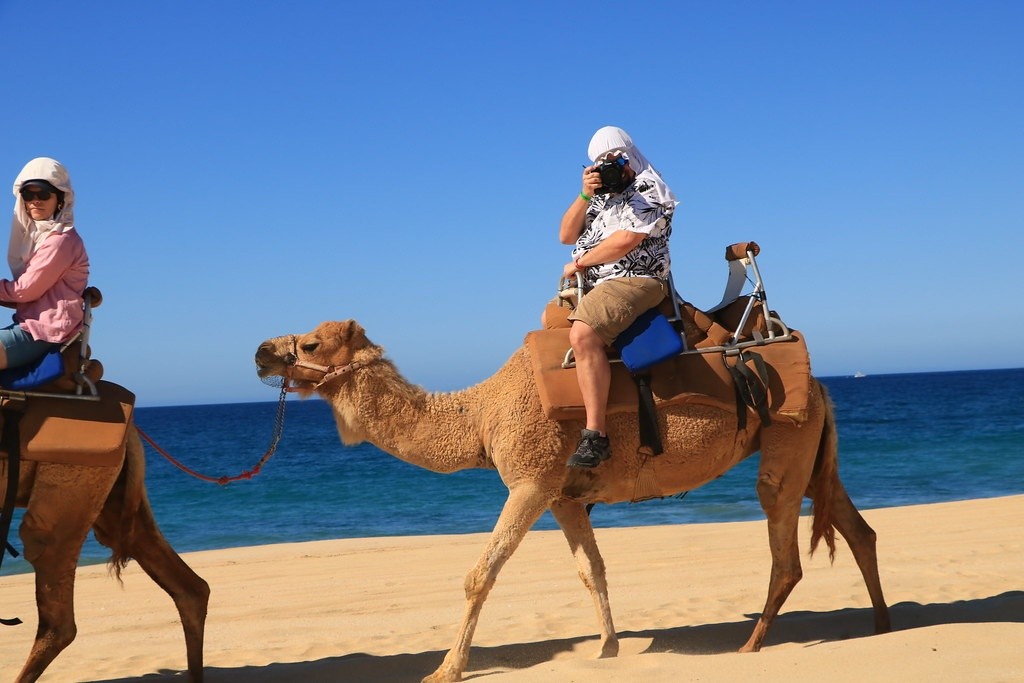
[18,179,58,193]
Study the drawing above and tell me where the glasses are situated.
[616,155,629,166]
[21,190,57,201]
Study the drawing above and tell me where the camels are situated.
[0,381,213,683]
[253,290,894,683]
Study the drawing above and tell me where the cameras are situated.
[590,159,624,194]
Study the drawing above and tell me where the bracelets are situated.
[580,192,591,201]
[575,258,584,270]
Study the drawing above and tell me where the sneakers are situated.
[567,429,612,470]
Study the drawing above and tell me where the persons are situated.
[0,157,92,367]
[559,124,680,468]
